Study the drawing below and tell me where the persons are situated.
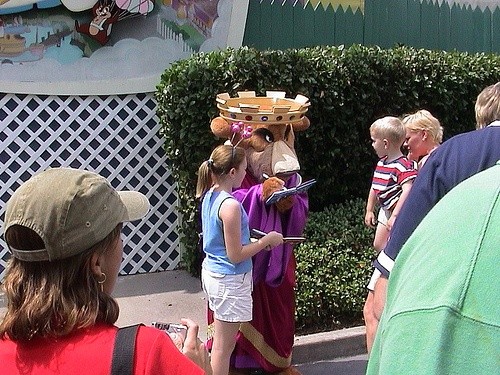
[0,168,213,375]
[364,82,500,375]
[196,145,283,375]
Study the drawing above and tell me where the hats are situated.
[3,166,151,261]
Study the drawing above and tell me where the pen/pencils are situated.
[262,174,286,190]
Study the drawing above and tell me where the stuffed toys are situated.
[208,91,310,375]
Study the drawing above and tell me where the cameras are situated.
[151,322,187,345]
